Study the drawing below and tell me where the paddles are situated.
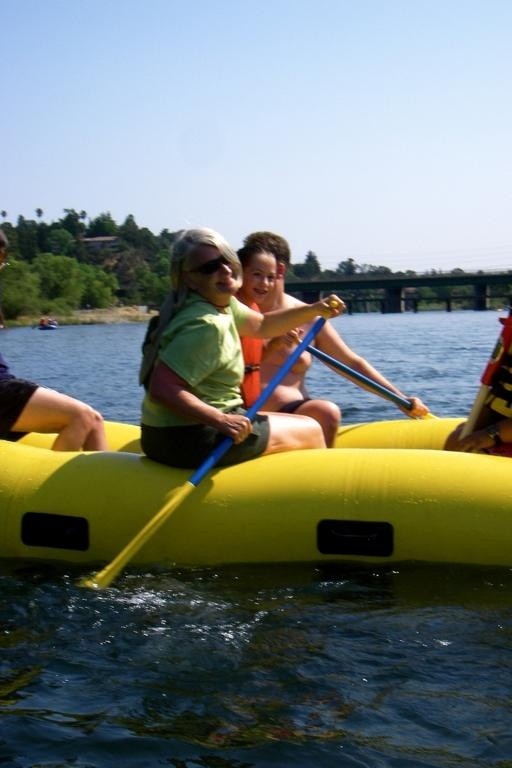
[87,302,332,593]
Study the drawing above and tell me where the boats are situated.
[0,411,512,575]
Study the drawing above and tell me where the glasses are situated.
[187,255,230,275]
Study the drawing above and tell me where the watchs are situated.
[486,419,503,448]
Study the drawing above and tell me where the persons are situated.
[232,243,278,410]
[242,230,431,448]
[138,226,348,471]
[442,305,512,459]
[38,317,58,326]
[0,229,108,451]
